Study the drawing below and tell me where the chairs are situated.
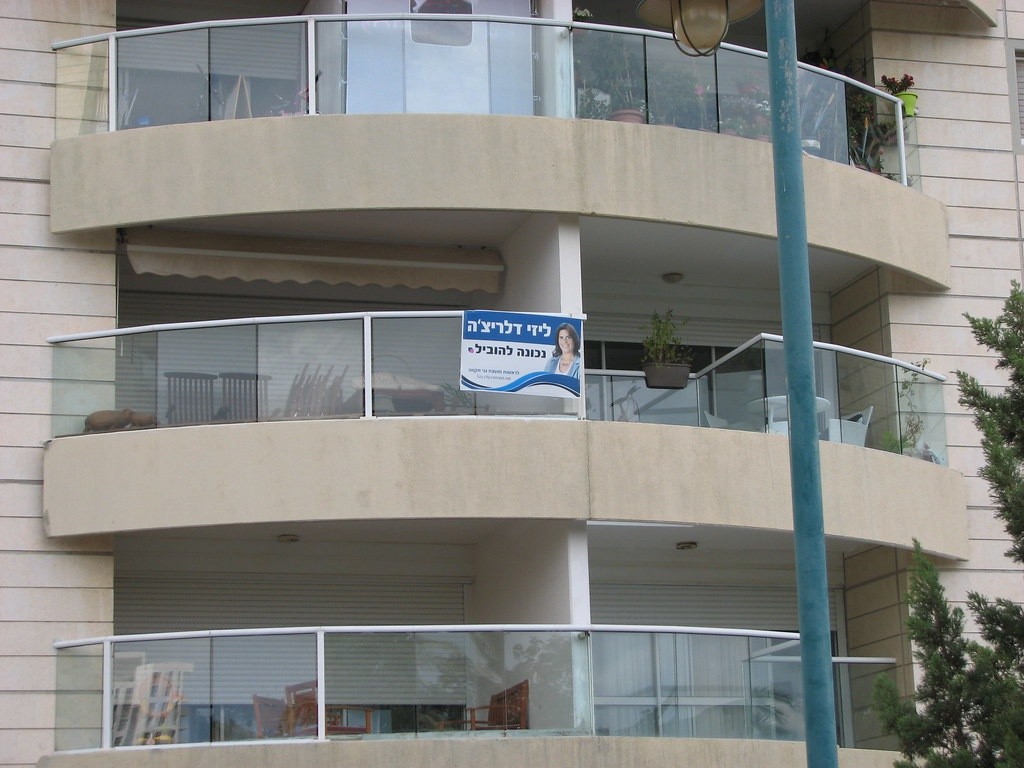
[219,372,271,421]
[438,679,528,731]
[163,372,218,424]
[285,681,374,737]
[819,406,874,446]
[703,410,750,430]
[253,693,288,738]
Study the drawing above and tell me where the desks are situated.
[342,387,446,414]
[744,395,832,434]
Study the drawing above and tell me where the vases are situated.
[869,167,881,175]
[750,135,770,142]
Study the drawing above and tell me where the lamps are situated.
[663,273,683,284]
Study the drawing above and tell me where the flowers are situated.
[679,66,770,135]
[867,149,885,170]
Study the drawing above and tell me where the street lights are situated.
[670,0,838,768]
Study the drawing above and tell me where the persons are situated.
[544,323,580,379]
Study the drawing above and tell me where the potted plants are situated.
[881,73,918,117]
[605,36,646,123]
[648,61,685,127]
[639,308,695,389]
[854,157,867,170]
[712,118,742,135]
